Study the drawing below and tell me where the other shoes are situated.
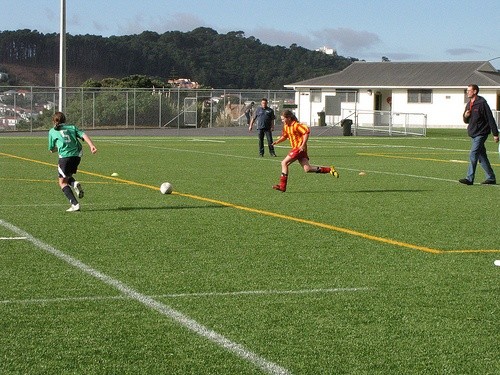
[271,153,276,157]
[481,179,496,184]
[259,155,263,157]
[459,178,473,185]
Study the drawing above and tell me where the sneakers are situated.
[66,203,79,212]
[330,165,339,178]
[272,185,286,192]
[74,181,84,199]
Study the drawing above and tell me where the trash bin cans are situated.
[317,111,327,127]
[340,119,353,136]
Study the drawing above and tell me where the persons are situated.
[244,102,255,127]
[269,110,339,192]
[48,111,98,212]
[249,97,277,157]
[457,84,499,185]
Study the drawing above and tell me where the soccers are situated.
[160,182,173,195]
